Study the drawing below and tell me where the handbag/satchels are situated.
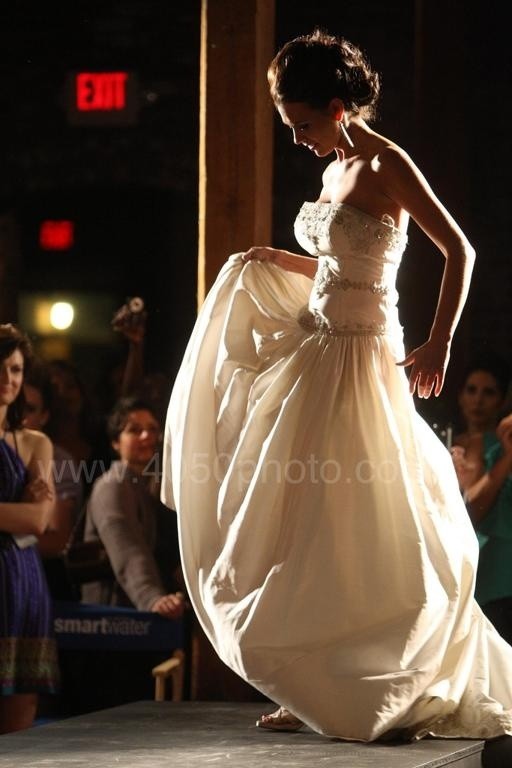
[62,473,142,583]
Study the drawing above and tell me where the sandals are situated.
[256,703,306,732]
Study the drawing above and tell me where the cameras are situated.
[109,296,145,329]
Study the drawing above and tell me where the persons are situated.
[159,27,510,742]
